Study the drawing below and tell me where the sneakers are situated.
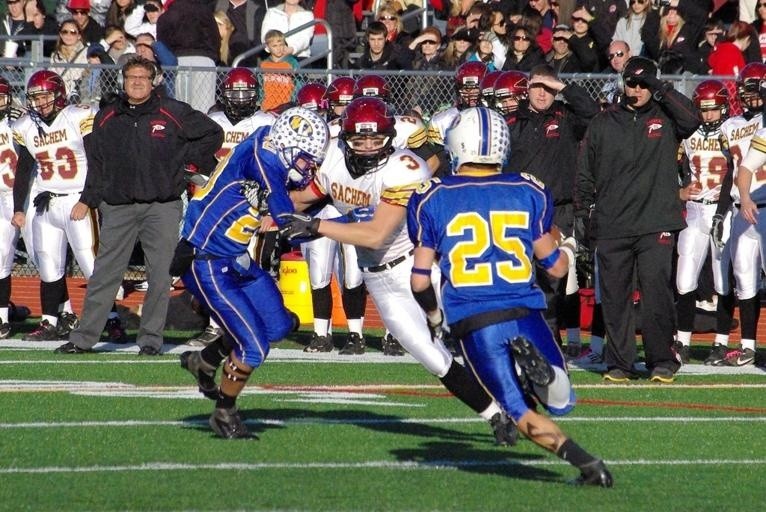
[564,341,689,383]
[509,335,555,387]
[138,346,161,356]
[705,342,760,368]
[0,302,126,353]
[382,332,404,356]
[568,469,613,488]
[339,333,366,355]
[184,326,224,346]
[489,412,519,447]
[209,408,258,442]
[304,332,334,352]
[179,351,220,400]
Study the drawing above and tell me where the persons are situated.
[177,96,516,446]
[2,2,766,383]
[406,106,614,488]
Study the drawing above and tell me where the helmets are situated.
[692,81,730,130]
[736,62,766,108]
[222,68,259,119]
[0,75,11,121]
[298,75,396,174]
[454,62,529,115]
[25,71,66,123]
[445,107,511,174]
[269,107,330,188]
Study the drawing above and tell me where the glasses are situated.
[60,30,78,35]
[421,0,645,60]
[379,17,397,20]
[626,81,649,89]
[758,3,766,8]
[71,10,87,14]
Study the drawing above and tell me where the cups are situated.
[4,41,18,60]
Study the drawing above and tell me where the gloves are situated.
[576,216,589,242]
[711,214,725,248]
[743,79,766,100]
[624,69,669,100]
[33,192,54,213]
[426,311,452,342]
[277,212,319,240]
[239,180,269,212]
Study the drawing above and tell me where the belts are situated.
[51,192,84,197]
[358,248,414,272]
[693,199,719,204]
[735,203,766,209]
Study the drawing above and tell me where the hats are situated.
[87,44,105,57]
[623,56,661,83]
[66,0,90,9]
